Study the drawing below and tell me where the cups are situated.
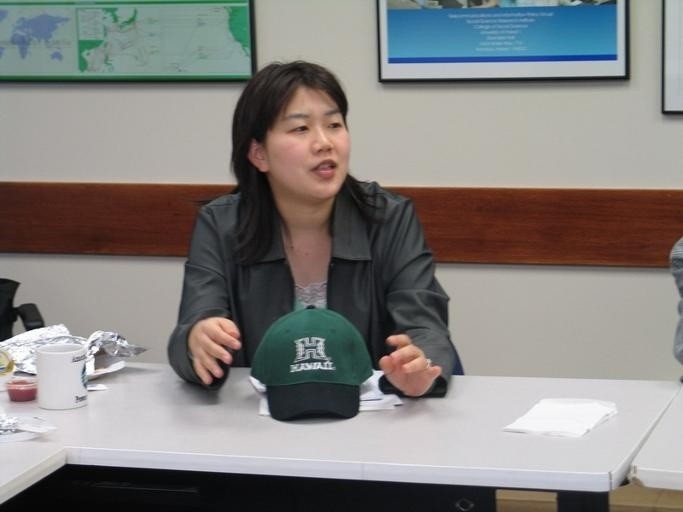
[34,342,90,411]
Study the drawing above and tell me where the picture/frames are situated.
[376,0,631,83]
[1,1,259,84]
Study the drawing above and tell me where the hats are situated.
[250,304,373,420]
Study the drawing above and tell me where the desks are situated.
[0,363,683,511]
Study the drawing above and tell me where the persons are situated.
[167,61,464,399]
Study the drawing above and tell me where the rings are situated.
[424,359,433,370]
